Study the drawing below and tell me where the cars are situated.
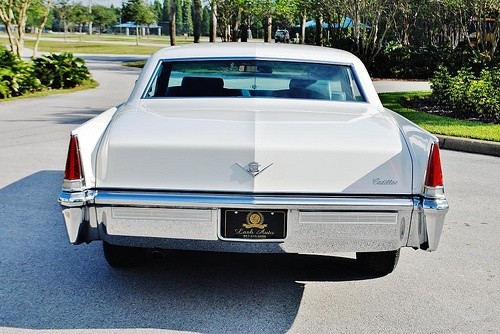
[58,40,448,276]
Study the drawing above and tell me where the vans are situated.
[275,30,290,43]
